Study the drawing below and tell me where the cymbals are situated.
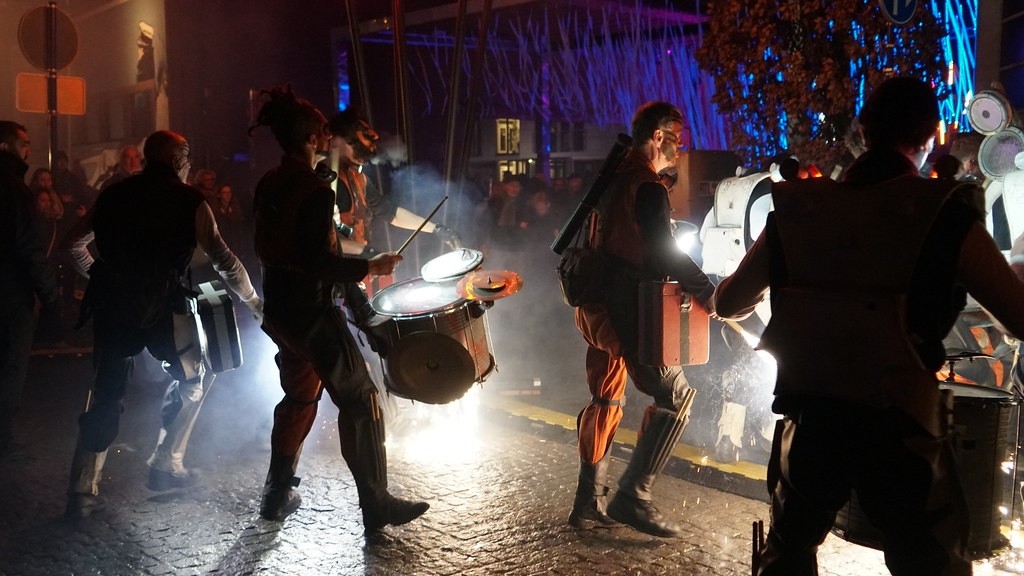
[456,268,523,301]
[385,331,475,403]
[945,346,998,362]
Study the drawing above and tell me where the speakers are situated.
[29,351,97,402]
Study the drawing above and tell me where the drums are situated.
[368,276,496,399]
[421,247,485,283]
[966,89,1024,182]
[829,379,1015,555]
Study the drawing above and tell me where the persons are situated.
[711,74,1024,576]
[0,119,264,521]
[319,111,464,436]
[253,98,430,534]
[555,99,725,538]
[475,172,589,262]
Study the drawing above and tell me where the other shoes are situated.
[145,466,202,491]
[609,492,681,538]
[568,505,625,531]
[64,493,108,522]
[362,497,429,536]
[260,489,303,521]
